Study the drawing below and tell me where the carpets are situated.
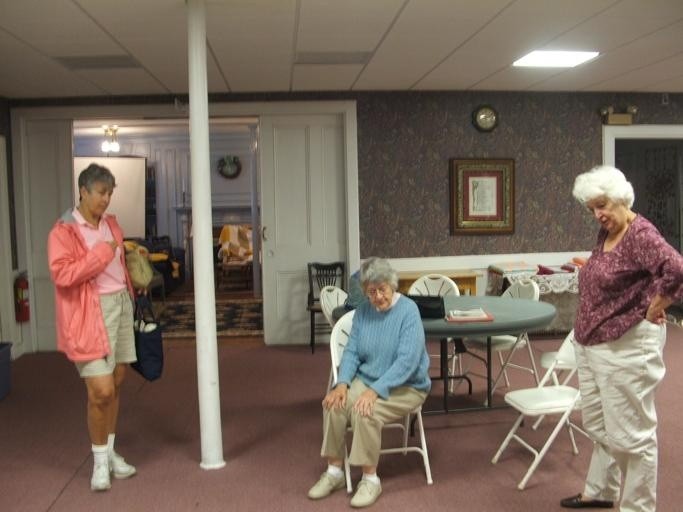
[144,298,263,339]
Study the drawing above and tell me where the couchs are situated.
[501,274,579,335]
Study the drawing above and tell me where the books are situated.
[445,314,494,323]
[448,308,488,320]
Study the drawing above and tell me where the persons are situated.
[560,165,683,512]
[308,257,432,508]
[47,163,148,491]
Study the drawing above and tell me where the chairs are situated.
[216,221,261,290]
[326,307,433,495]
[133,262,165,307]
[320,284,349,327]
[491,327,598,490]
[452,276,540,408]
[303,260,345,353]
[405,274,462,394]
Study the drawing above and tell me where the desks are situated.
[422,296,558,417]
[484,265,536,296]
[397,267,482,295]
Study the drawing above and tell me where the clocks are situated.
[471,104,498,132]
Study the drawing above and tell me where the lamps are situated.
[99,124,121,153]
[599,103,639,116]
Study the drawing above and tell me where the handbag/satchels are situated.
[126,245,153,289]
[406,295,445,318]
[130,319,163,382]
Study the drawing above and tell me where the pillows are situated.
[536,265,554,275]
[560,263,578,273]
[571,256,586,267]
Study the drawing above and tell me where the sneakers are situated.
[350,476,382,508]
[307,471,345,499]
[91,459,113,491]
[109,449,136,479]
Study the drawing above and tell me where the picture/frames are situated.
[448,157,515,235]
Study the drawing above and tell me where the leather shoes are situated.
[560,493,613,508]
